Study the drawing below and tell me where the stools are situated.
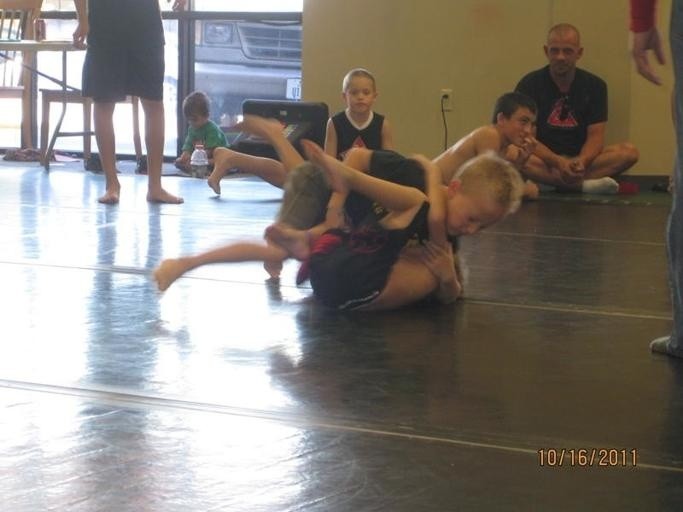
[39,88,142,166]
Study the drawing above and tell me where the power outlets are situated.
[441,89,453,111]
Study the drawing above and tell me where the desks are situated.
[0,38,87,149]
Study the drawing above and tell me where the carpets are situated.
[532,187,672,206]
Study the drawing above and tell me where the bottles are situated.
[188,144,209,178]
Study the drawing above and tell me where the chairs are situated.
[0,0,42,149]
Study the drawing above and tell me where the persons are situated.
[630,0,680,358]
[74,0,188,204]
[154,146,523,294]
[505,22,640,195]
[205,90,538,203]
[174,91,227,168]
[322,68,394,163]
[261,138,460,314]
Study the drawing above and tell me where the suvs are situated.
[192,19,302,126]
